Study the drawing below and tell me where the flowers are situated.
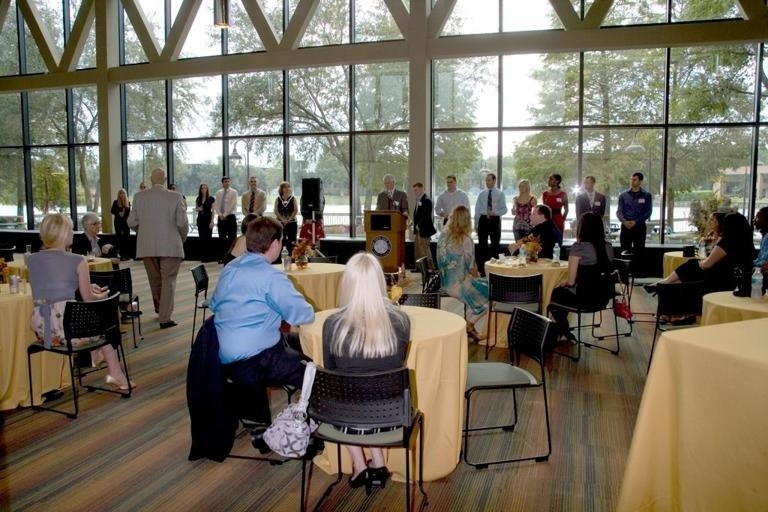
[515,234,542,258]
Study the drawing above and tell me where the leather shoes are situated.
[125,310,143,317]
[159,320,178,329]
[120,312,136,322]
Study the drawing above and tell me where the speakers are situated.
[301,177,324,212]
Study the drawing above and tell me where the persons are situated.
[576,176,606,221]
[196,177,326,269]
[617,173,653,279]
[513,176,568,258]
[375,175,409,217]
[476,175,506,276]
[438,206,488,341]
[551,212,614,341]
[72,168,189,330]
[413,176,471,272]
[209,216,315,391]
[322,252,410,497]
[27,213,136,390]
[644,207,768,327]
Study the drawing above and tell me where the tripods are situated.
[311,211,327,258]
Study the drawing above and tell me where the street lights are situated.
[228,140,250,194]
[137,140,155,190]
[626,127,652,221]
[475,156,491,194]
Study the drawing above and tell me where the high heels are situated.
[567,332,579,344]
[104,374,137,392]
[367,460,391,490]
[643,285,659,297]
[348,467,373,496]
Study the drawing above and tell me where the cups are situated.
[87,251,96,263]
[283,256,292,272]
[683,246,695,258]
[17,279,28,296]
[498,253,505,263]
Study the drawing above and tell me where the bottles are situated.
[519,244,527,264]
[699,236,707,257]
[693,237,699,257]
[750,268,763,300]
[552,242,561,264]
[9,275,17,294]
[24,244,33,268]
[281,246,290,268]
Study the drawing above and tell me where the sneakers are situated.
[467,322,481,342]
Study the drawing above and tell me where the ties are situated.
[487,189,492,212]
[249,191,255,214]
[387,195,392,210]
[220,190,227,214]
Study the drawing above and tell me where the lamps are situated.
[214,0,231,28]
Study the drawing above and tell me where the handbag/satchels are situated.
[613,298,632,320]
[261,401,318,458]
